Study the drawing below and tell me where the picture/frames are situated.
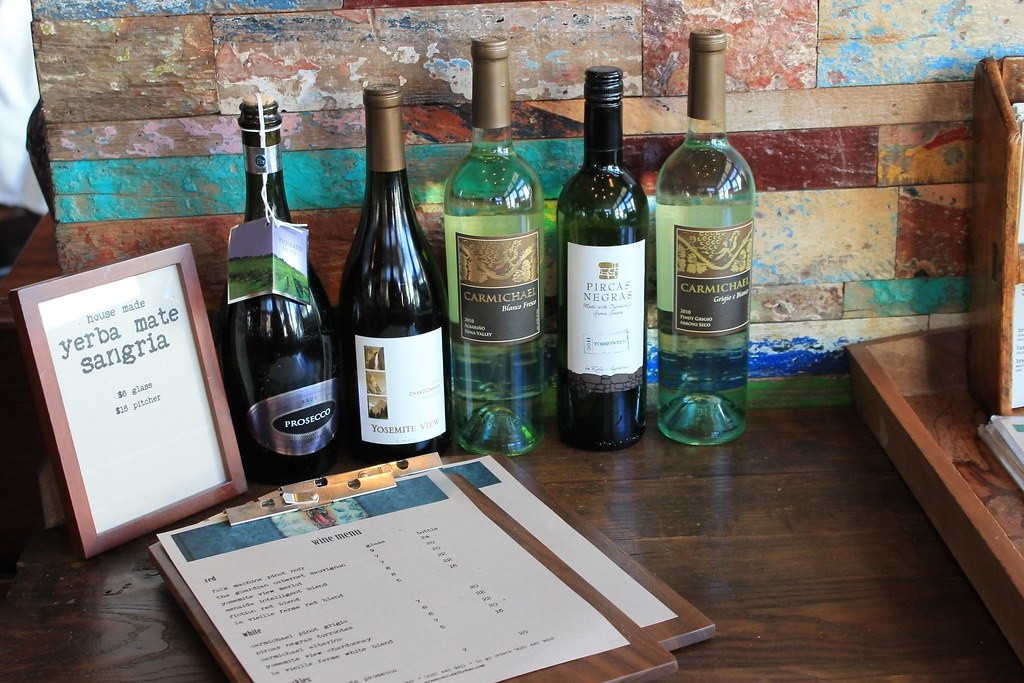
[9,243,247,562]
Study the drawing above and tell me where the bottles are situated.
[218,29,755,486]
